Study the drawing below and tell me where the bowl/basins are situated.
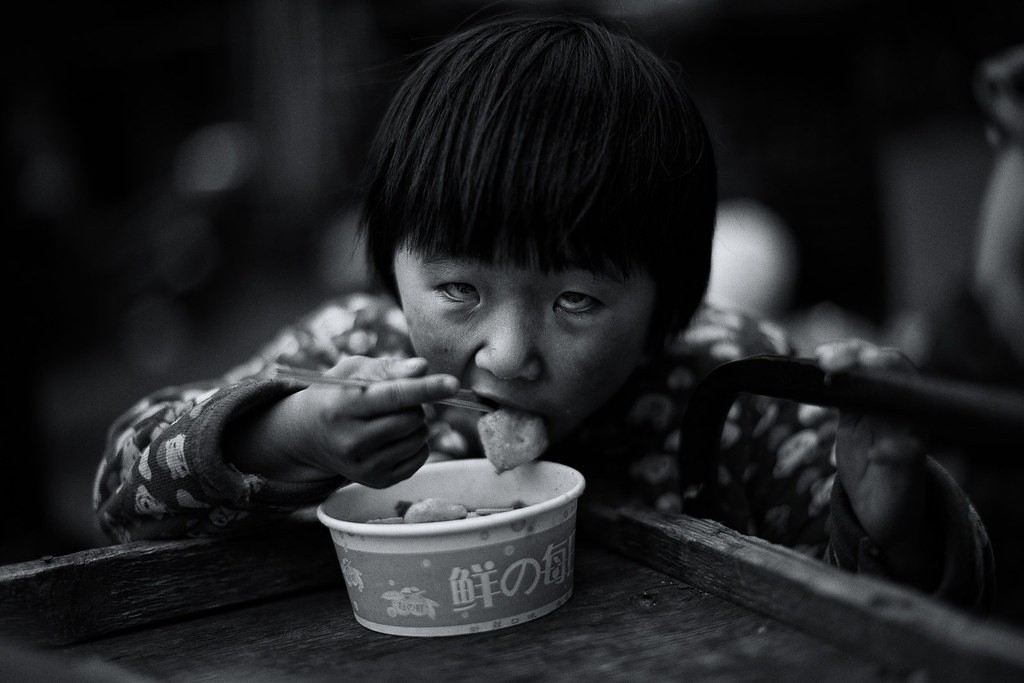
[317,457,585,637]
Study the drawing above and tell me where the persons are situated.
[92,12,994,618]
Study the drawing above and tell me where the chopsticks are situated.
[272,364,497,414]
